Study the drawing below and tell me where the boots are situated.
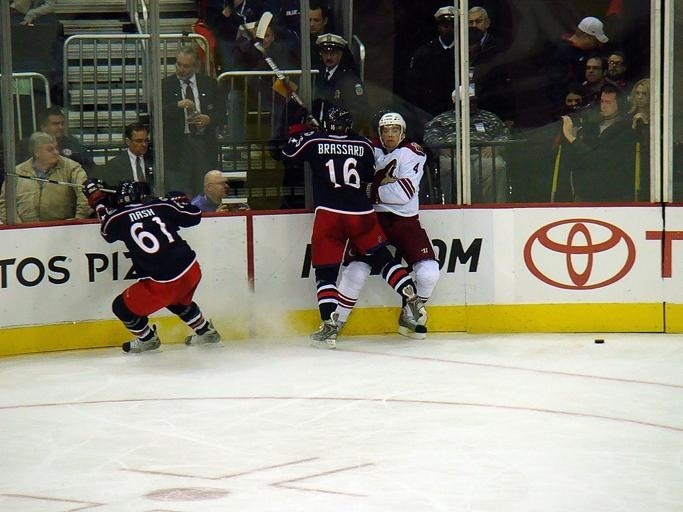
[402,285,428,325]
[184,318,222,346]
[122,324,161,353]
[399,307,427,333]
[308,312,340,351]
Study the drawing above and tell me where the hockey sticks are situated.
[236,2,319,128]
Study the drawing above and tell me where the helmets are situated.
[377,112,408,149]
[323,107,354,136]
[115,181,152,206]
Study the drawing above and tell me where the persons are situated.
[317,112,440,338]
[81,176,226,353]
[269,94,429,349]
[0,131,95,225]
[89,122,154,219]
[161,0,659,212]
[15,107,96,173]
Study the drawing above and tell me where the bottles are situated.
[191,110,204,132]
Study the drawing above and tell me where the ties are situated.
[185,82,198,134]
[136,156,145,184]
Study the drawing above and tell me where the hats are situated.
[577,16,609,44]
[451,86,476,102]
[434,6,460,20]
[315,34,349,51]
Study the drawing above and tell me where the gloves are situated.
[165,191,190,209]
[82,180,104,208]
